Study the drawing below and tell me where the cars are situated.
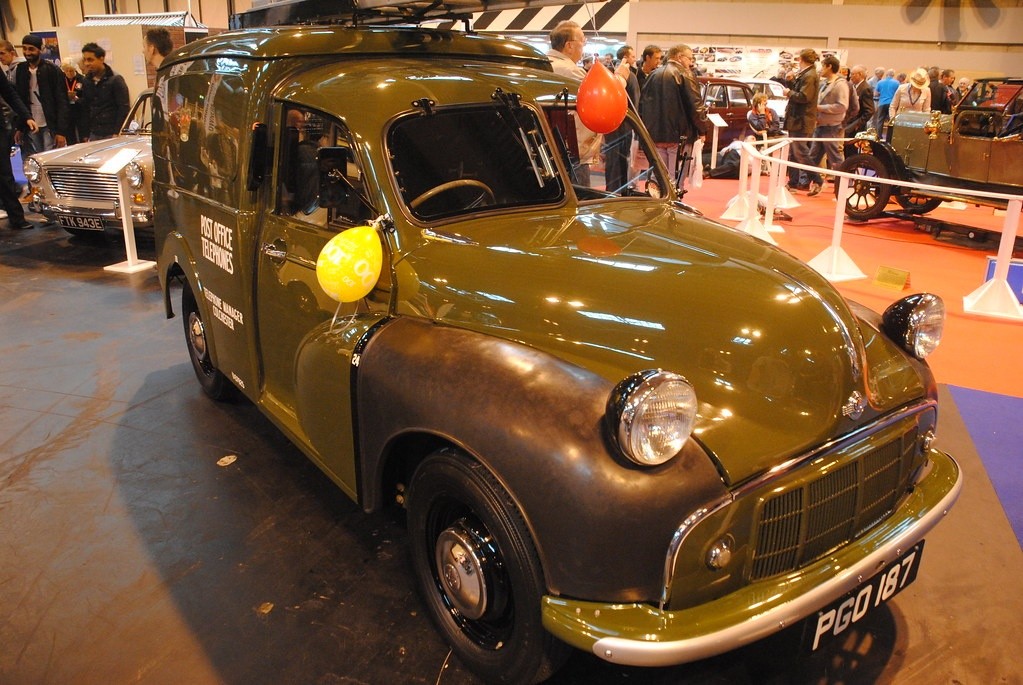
[676,75,770,162]
[701,74,793,129]
[21,87,159,243]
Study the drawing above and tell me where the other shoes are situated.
[0,209,8,218]
[785,183,798,193]
[17,221,32,229]
[20,193,34,204]
[702,170,708,179]
[807,183,826,196]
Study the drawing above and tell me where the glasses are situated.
[680,54,692,62]
[569,38,588,45]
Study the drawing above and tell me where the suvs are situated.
[832,76,1023,221]
[145,23,966,684]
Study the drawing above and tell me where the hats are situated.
[909,67,930,90]
[22,35,42,50]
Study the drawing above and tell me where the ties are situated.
[854,85,856,88]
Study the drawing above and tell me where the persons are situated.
[548,21,631,189]
[770,49,991,197]
[153,109,324,215]
[702,93,780,179]
[582,44,711,198]
[0,36,131,229]
[143,27,174,68]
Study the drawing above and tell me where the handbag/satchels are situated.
[688,139,705,189]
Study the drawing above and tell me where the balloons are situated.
[316,226,384,303]
[576,62,629,133]
